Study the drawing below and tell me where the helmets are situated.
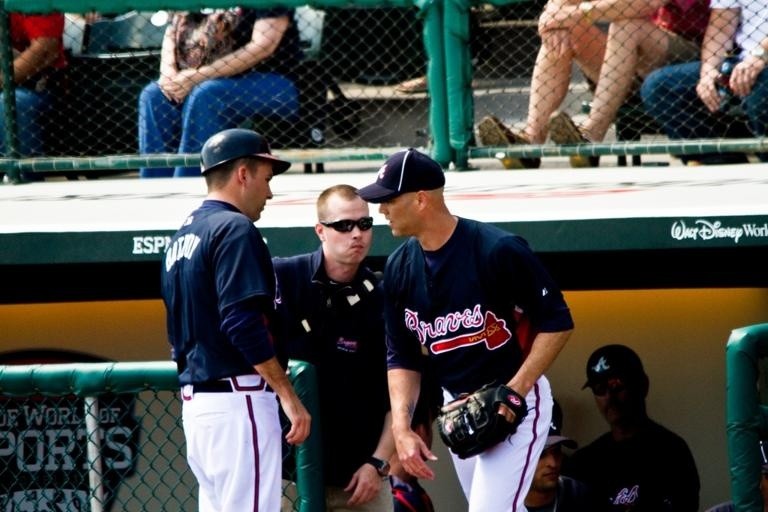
[580,343,644,391]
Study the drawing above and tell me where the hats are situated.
[541,433,581,456]
[354,148,445,204]
[199,128,291,177]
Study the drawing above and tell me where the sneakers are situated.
[547,111,598,167]
[477,116,541,169]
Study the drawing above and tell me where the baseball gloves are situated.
[438,381,527,460]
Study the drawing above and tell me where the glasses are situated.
[320,216,373,233]
[714,56,741,101]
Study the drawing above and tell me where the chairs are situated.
[580,75,752,166]
[54,11,322,155]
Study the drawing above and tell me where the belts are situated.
[193,376,274,394]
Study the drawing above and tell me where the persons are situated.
[522,344,700,511]
[473,0,768,168]
[270,184,400,512]
[353,148,575,511]
[4,6,431,180]
[161,129,311,512]
[386,421,434,512]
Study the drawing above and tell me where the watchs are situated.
[367,457,391,476]
